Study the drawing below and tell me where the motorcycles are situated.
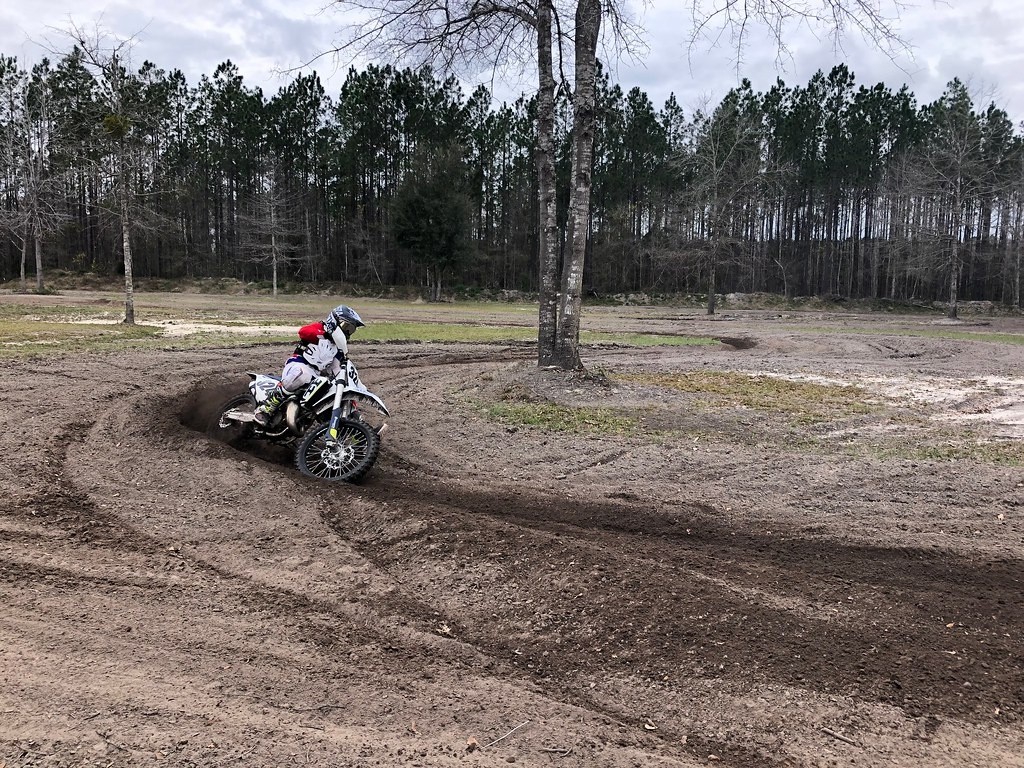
[206,322,390,485]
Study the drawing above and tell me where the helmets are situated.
[326,303,365,340]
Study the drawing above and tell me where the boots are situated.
[253,381,295,427]
[374,423,389,440]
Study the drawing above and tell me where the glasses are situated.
[342,320,356,337]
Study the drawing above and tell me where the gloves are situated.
[327,311,340,333]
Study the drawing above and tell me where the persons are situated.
[254,305,366,426]
